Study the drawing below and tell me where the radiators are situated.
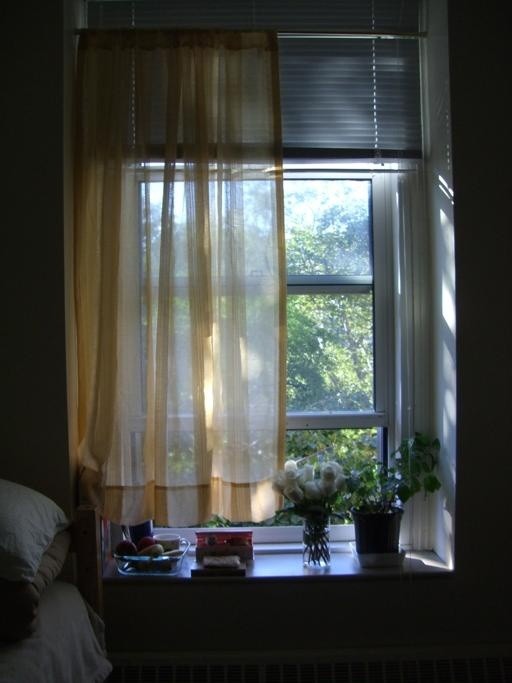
[104,645,512,683]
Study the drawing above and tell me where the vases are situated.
[301,516,332,568]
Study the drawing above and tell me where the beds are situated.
[0,478,113,683]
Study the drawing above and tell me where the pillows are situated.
[0,479,70,583]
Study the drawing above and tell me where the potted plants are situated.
[345,431,442,566]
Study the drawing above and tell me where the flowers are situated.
[270,457,346,566]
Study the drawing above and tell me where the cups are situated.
[152,533,190,552]
